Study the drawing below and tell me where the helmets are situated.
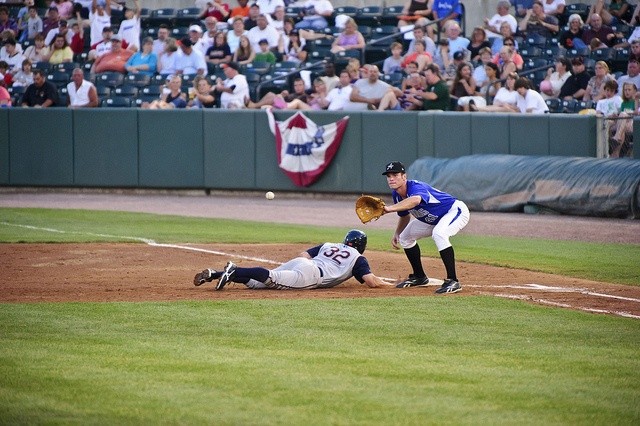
[344,229,367,253]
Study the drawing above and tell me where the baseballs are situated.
[266,192,274,200]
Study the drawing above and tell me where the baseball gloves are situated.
[355,195,385,224]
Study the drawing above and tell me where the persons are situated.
[381,161,471,294]
[193,229,395,291]
[597,79,639,158]
[2,0,640,112]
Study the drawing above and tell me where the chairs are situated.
[169,27,187,43]
[244,61,271,81]
[139,85,162,97]
[52,61,78,75]
[436,3,468,45]
[75,52,89,61]
[363,45,388,62]
[563,100,574,112]
[213,60,238,76]
[54,89,68,106]
[112,84,138,96]
[96,70,125,86]
[242,72,260,97]
[150,8,175,27]
[365,27,398,42]
[137,5,150,25]
[285,6,304,21]
[181,73,199,81]
[47,72,70,87]
[143,27,157,43]
[332,4,359,23]
[524,24,546,47]
[151,73,170,86]
[361,5,381,22]
[594,47,612,68]
[308,37,332,51]
[96,85,111,98]
[7,86,26,104]
[335,49,359,71]
[322,24,339,36]
[548,34,560,48]
[82,61,95,77]
[178,8,201,26]
[577,99,592,112]
[37,6,50,16]
[261,74,277,94]
[380,70,404,87]
[384,3,406,23]
[615,48,630,68]
[523,58,547,78]
[521,47,540,61]
[181,82,194,93]
[570,48,590,69]
[545,47,565,66]
[305,49,332,74]
[357,24,371,38]
[8,5,20,17]
[126,73,150,86]
[131,96,158,108]
[559,2,588,20]
[545,97,558,110]
[102,96,130,106]
[31,62,48,74]
[274,62,297,80]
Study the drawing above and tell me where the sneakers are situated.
[438,279,462,292]
[217,261,238,290]
[193,268,215,286]
[396,274,429,287]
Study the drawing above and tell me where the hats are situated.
[189,23,203,34]
[382,161,405,175]
[2,38,15,45]
[176,38,191,48]
